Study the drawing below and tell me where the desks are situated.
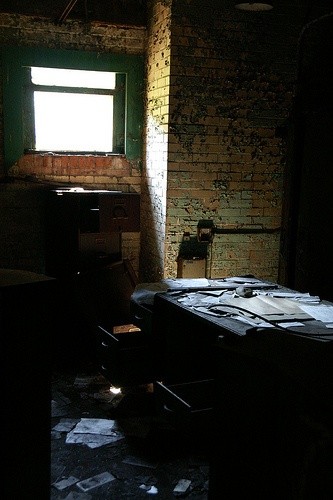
[153,277,331,432]
